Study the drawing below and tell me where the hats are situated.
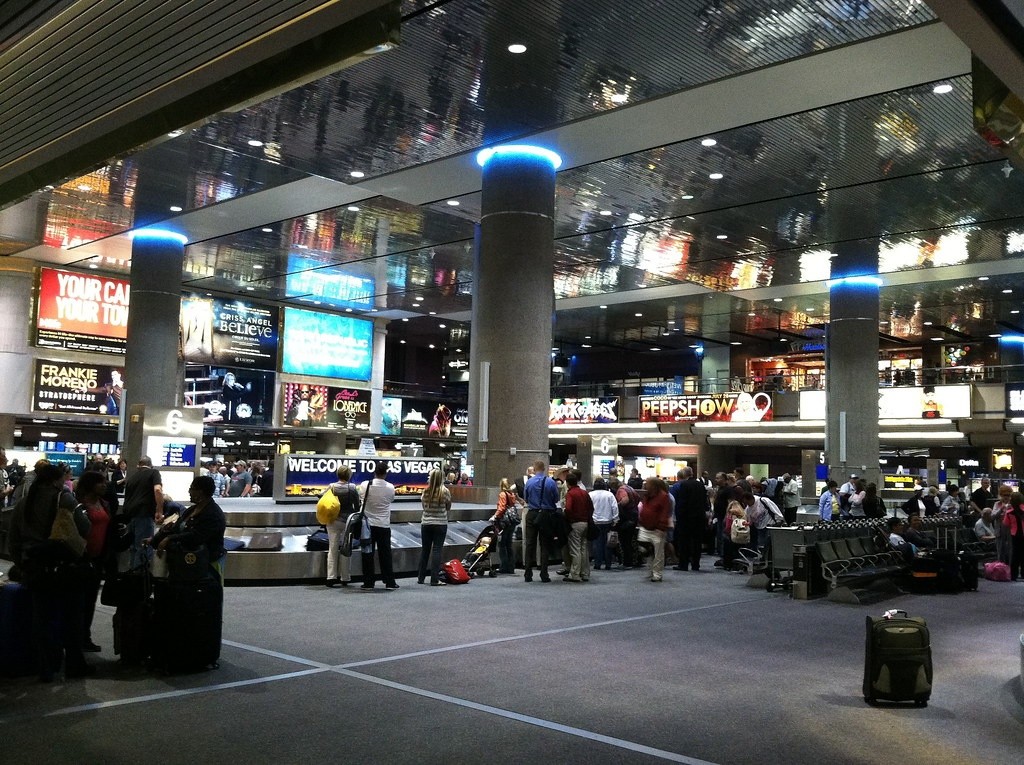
[234,460,247,468]
[849,473,859,479]
[914,485,924,491]
[783,473,791,479]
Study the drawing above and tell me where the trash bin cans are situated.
[793,544,821,600]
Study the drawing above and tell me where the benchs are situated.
[733,537,774,589]
[816,525,999,606]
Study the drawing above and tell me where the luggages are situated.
[864,608,932,710]
[940,548,979,595]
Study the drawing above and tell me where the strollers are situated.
[460,519,508,579]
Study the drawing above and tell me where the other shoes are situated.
[324,553,734,592]
[83,640,103,653]
[70,652,94,676]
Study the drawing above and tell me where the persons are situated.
[286,384,325,426]
[921,387,944,418]
[731,393,760,421]
[221,372,246,424]
[418,468,451,585]
[461,537,492,568]
[111,459,128,493]
[201,460,274,497]
[819,473,887,523]
[70,367,125,415]
[444,463,472,485]
[429,403,452,437]
[754,370,784,391]
[0,446,113,655]
[122,455,163,571]
[895,368,917,385]
[162,493,188,517]
[141,477,228,567]
[697,468,802,572]
[317,462,400,589]
[488,461,710,582]
[889,477,1024,591]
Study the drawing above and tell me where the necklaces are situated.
[93,501,101,512]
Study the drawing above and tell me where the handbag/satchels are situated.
[607,526,618,549]
[894,541,916,563]
[221,526,246,551]
[494,489,520,531]
[983,560,1012,582]
[145,547,168,577]
[773,519,784,527]
[247,531,284,551]
[315,482,340,526]
[439,559,471,585]
[351,513,362,540]
[118,542,148,576]
[47,490,89,559]
[534,509,556,528]
[306,528,330,551]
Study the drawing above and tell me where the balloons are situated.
[943,347,970,366]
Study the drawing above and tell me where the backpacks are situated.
[731,518,750,543]
